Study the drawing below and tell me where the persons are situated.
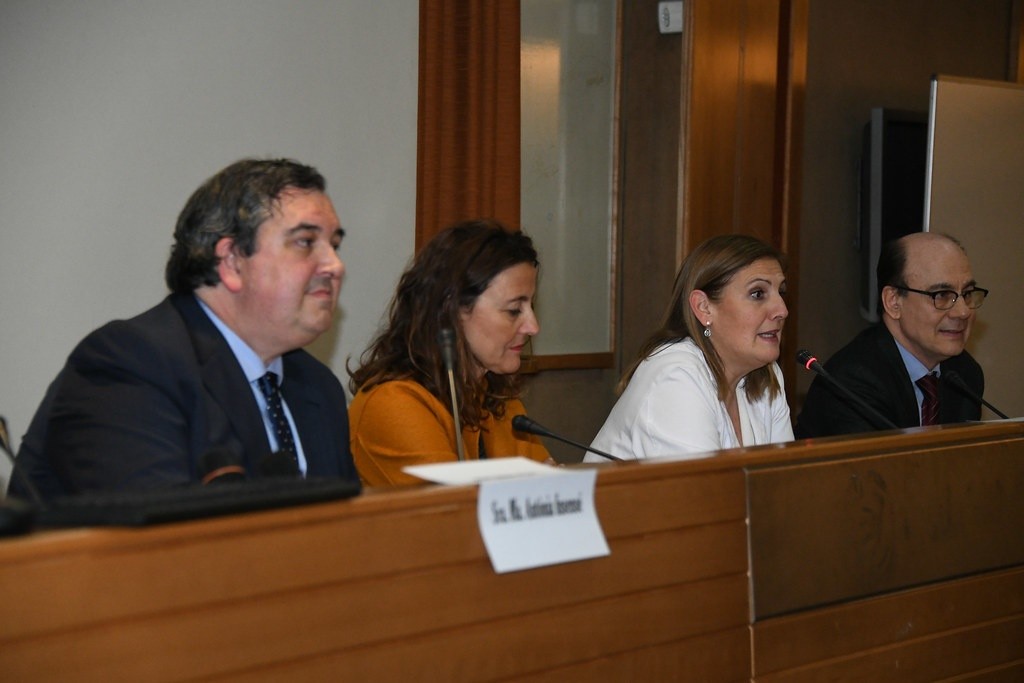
[7,158,360,505]
[797,232,989,441]
[582,233,795,463]
[347,221,567,492]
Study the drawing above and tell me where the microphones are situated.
[796,350,899,430]
[435,329,466,460]
[944,371,1009,420]
[204,447,248,485]
[511,416,624,463]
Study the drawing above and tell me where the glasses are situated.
[888,283,989,310]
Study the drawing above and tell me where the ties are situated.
[915,373,945,426]
[257,371,299,472]
